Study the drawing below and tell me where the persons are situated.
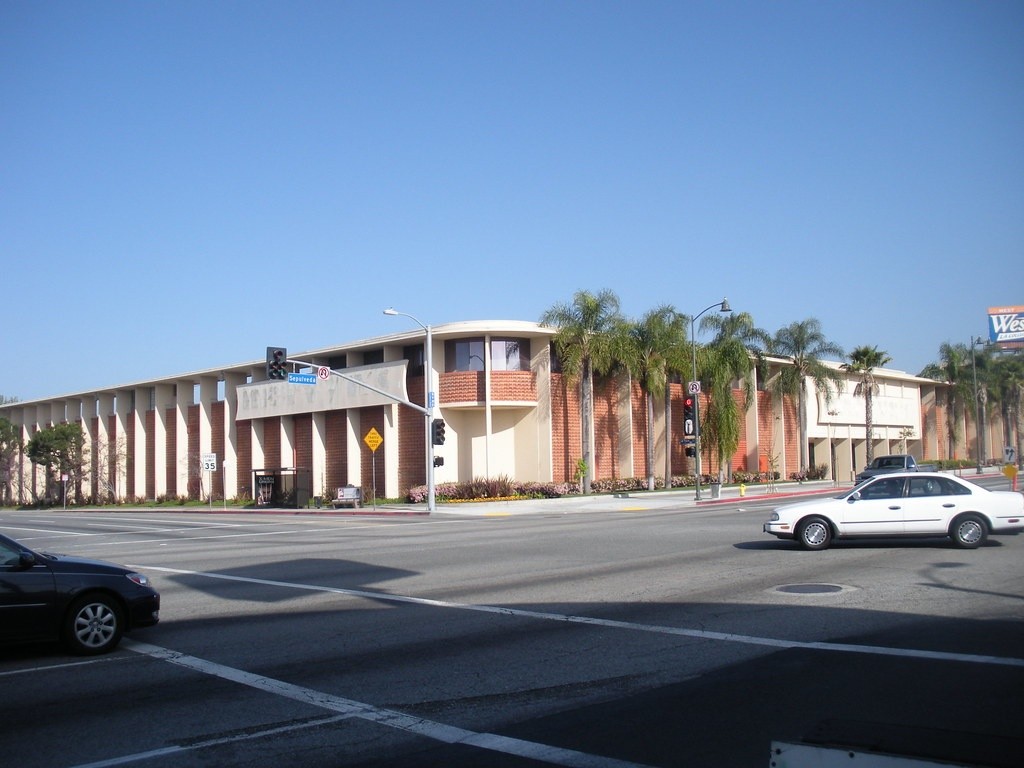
[885,479,899,498]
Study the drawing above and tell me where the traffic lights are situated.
[433,418,445,446]
[266,347,288,381]
[684,395,695,422]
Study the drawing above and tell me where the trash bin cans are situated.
[313,496,322,508]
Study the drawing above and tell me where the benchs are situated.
[332,488,363,509]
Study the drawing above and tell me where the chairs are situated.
[886,461,891,465]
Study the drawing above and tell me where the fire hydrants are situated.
[740,483,747,497]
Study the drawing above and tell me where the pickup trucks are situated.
[854,455,936,498]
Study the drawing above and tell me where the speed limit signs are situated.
[202,453,216,471]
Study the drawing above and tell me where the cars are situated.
[0,532,160,659]
[762,471,1024,551]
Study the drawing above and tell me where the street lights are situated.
[970,334,984,474]
[383,306,435,512]
[692,296,732,501]
